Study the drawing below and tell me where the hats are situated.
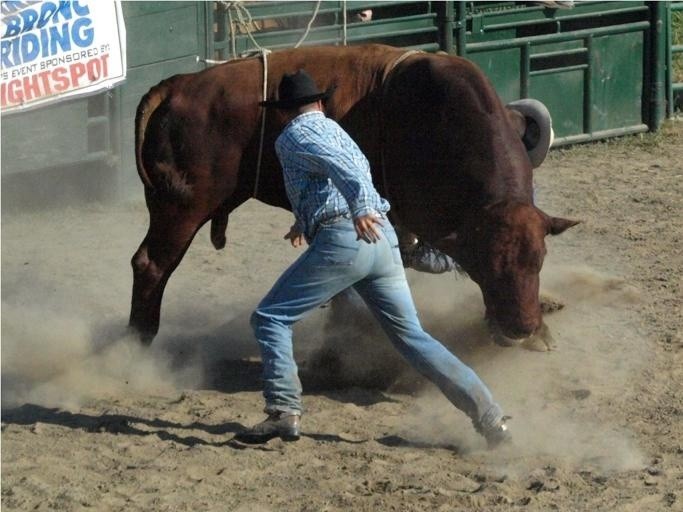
[259,69,337,110]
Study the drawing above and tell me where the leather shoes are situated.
[487,424,512,450]
[234,414,300,444]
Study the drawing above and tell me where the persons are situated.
[234,69,512,450]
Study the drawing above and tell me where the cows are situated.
[127,41,585,349]
